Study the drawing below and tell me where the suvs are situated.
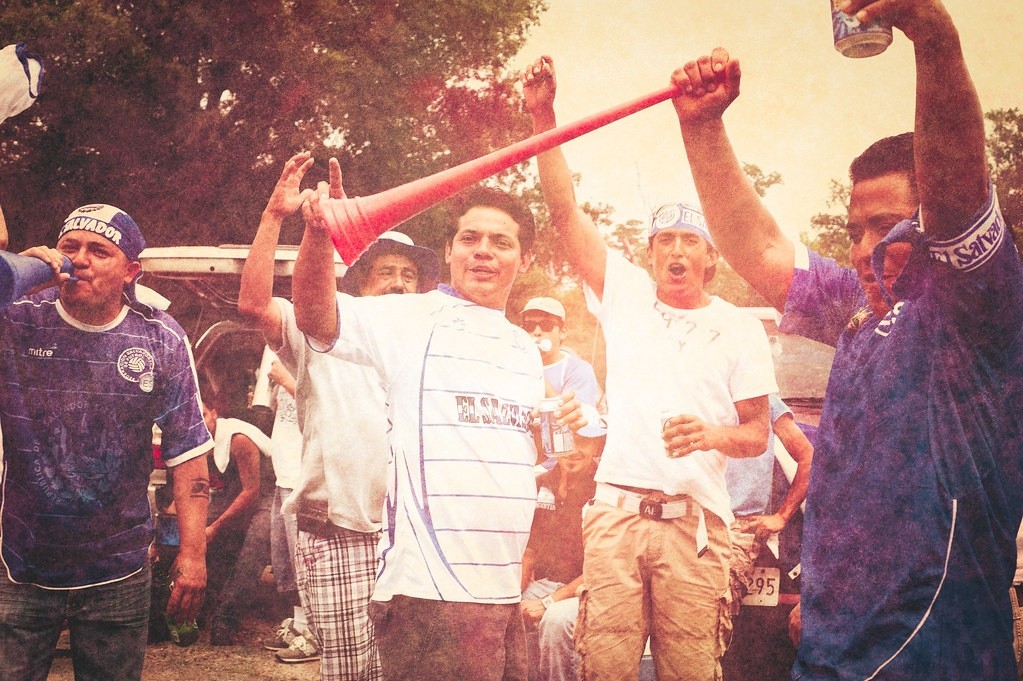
[738,309,839,606]
[120,244,358,520]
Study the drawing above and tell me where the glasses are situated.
[523,320,562,334]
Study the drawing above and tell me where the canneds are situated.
[662,409,693,458]
[830,0,893,57]
[539,397,576,457]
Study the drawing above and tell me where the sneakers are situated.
[274,629,321,663]
[263,620,303,650]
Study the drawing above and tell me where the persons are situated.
[153,150,821,681]
[669,0,1023,681]
[291,157,589,681]
[524,53,779,681]
[0,203,216,681]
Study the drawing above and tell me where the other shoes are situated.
[210,620,232,647]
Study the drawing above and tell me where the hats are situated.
[57,203,145,262]
[339,230,440,297]
[519,296,566,327]
[648,201,719,283]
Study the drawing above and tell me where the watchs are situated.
[542,595,553,610]
